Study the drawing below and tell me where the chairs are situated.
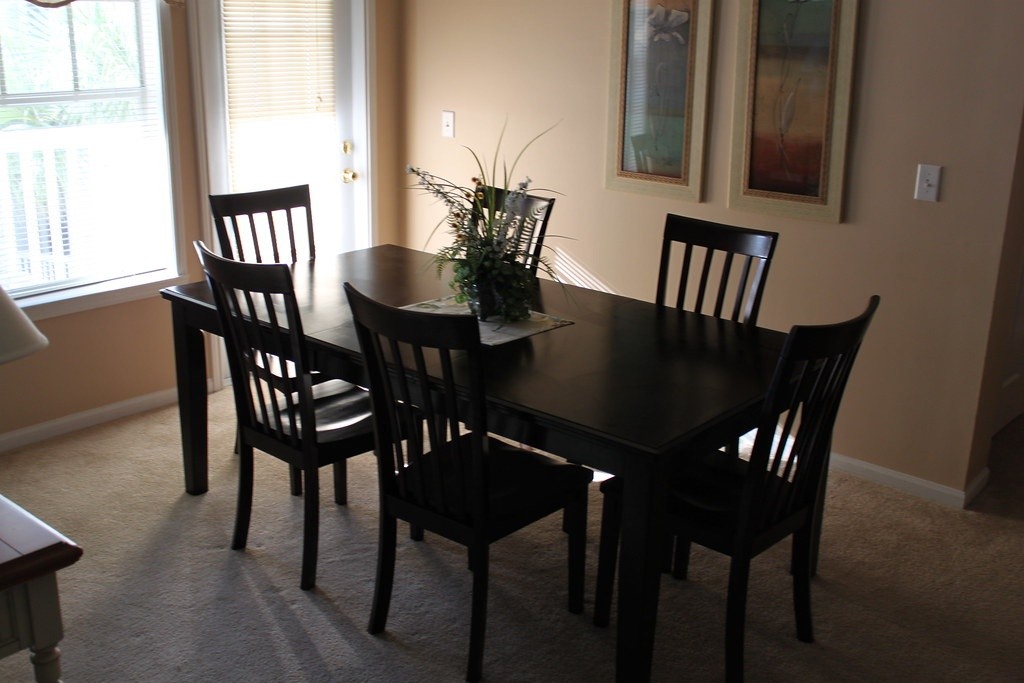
[562,211,781,578]
[342,279,595,681]
[209,182,316,452]
[591,292,883,683]
[194,239,428,593]
[432,182,553,440]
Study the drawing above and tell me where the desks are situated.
[1,494,84,683]
[161,244,846,683]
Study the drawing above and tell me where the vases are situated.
[462,278,502,320]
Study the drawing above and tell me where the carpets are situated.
[0,374,1024,683]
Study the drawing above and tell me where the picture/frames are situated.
[602,0,715,204]
[726,0,861,223]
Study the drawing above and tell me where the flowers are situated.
[406,107,586,330]
